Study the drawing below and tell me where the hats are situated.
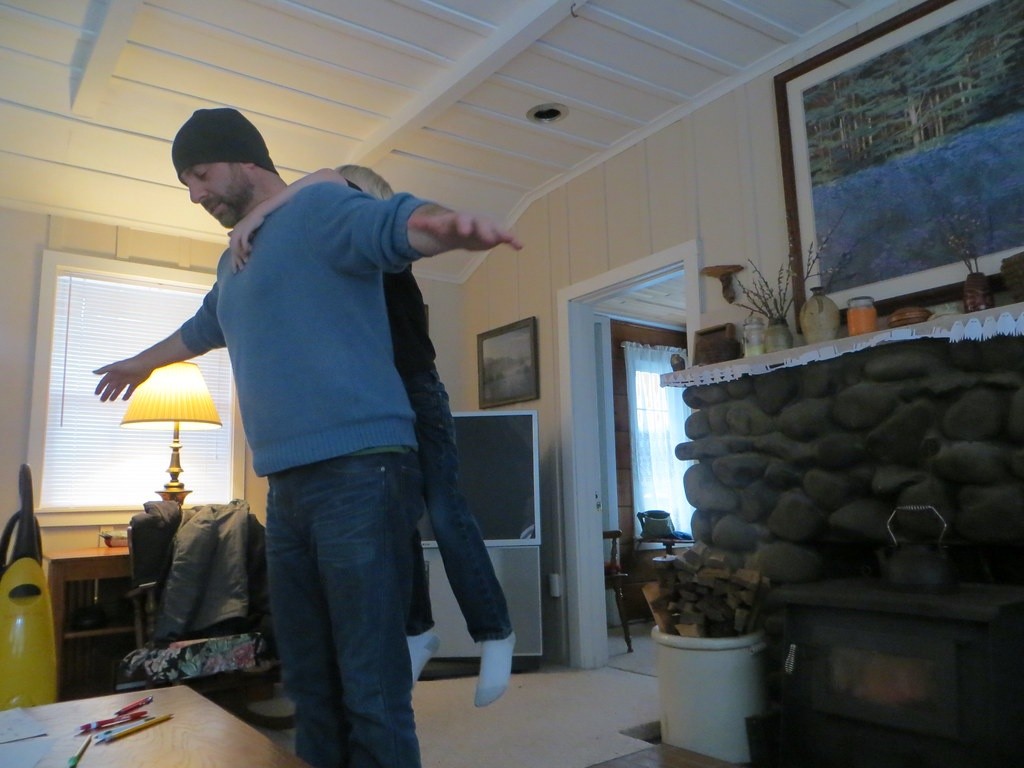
[172,108,280,187]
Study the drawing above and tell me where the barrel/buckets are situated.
[654,624,777,765]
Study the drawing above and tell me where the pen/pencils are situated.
[105,713,176,742]
[94,716,155,740]
[75,711,148,732]
[68,734,92,768]
[114,695,154,715]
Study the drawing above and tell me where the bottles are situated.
[846,296,878,337]
[742,317,766,357]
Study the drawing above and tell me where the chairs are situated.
[125,498,282,701]
[603,530,634,653]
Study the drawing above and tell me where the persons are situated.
[227,166,516,706]
[92,109,523,768]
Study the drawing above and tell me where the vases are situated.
[965,272,994,313]
[764,319,795,352]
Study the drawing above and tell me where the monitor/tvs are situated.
[416,410,541,546]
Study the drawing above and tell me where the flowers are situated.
[936,210,989,275]
[731,196,867,322]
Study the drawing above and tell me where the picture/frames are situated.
[775,0,1024,334]
[475,315,537,409]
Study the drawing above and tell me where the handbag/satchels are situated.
[637,510,675,538]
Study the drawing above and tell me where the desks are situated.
[639,536,695,555]
[1,684,321,767]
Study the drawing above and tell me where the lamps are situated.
[120,360,223,507]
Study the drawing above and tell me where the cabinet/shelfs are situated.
[41,548,143,701]
[420,546,543,664]
